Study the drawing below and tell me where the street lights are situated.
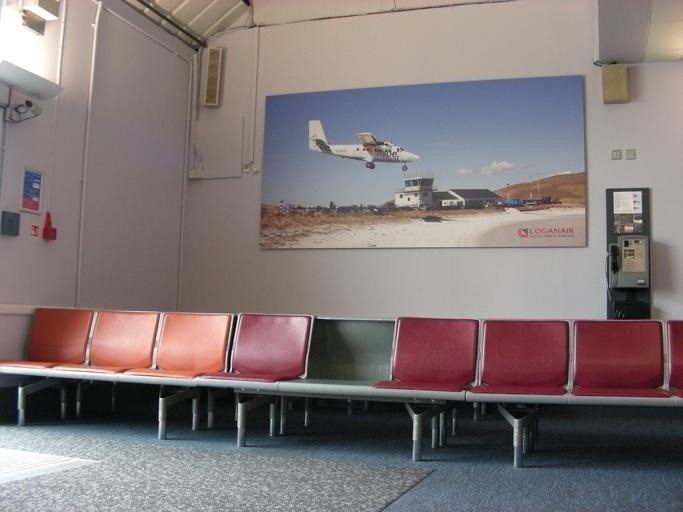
[505,183,509,200]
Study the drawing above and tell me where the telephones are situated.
[609,235,649,289]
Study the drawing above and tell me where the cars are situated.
[317,204,418,215]
[497,196,561,207]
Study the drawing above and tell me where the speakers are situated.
[203,47,222,107]
[602,63,630,104]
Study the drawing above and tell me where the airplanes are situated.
[306,118,421,171]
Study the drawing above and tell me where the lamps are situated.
[21,0,59,36]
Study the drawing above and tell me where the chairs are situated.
[0,308,683,468]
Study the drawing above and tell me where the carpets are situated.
[0,425,436,512]
